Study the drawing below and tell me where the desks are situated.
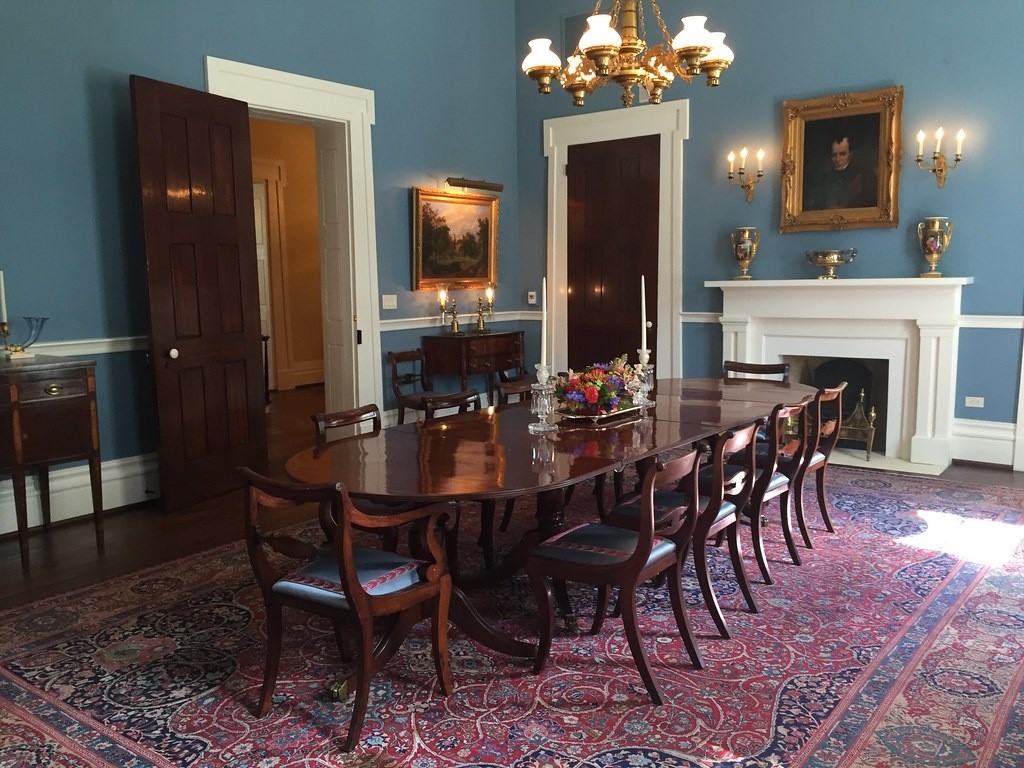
[288,378,822,705]
[420,330,526,402]
[0,355,108,587]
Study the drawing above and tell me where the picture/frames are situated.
[410,185,501,293]
[780,86,904,233]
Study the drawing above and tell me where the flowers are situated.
[558,359,644,413]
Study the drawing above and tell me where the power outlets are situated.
[965,396,984,408]
[528,291,537,305]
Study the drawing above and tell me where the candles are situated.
[482,281,497,304]
[540,277,548,368]
[640,275,647,350]
[0,271,8,326]
[434,282,450,305]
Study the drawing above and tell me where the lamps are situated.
[913,125,964,186]
[521,0,734,106]
[728,146,767,201]
[444,177,504,192]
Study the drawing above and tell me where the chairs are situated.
[498,360,847,707]
[386,348,481,425]
[313,403,460,571]
[422,393,482,416]
[242,466,453,752]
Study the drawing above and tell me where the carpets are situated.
[0,463,1024,768]
